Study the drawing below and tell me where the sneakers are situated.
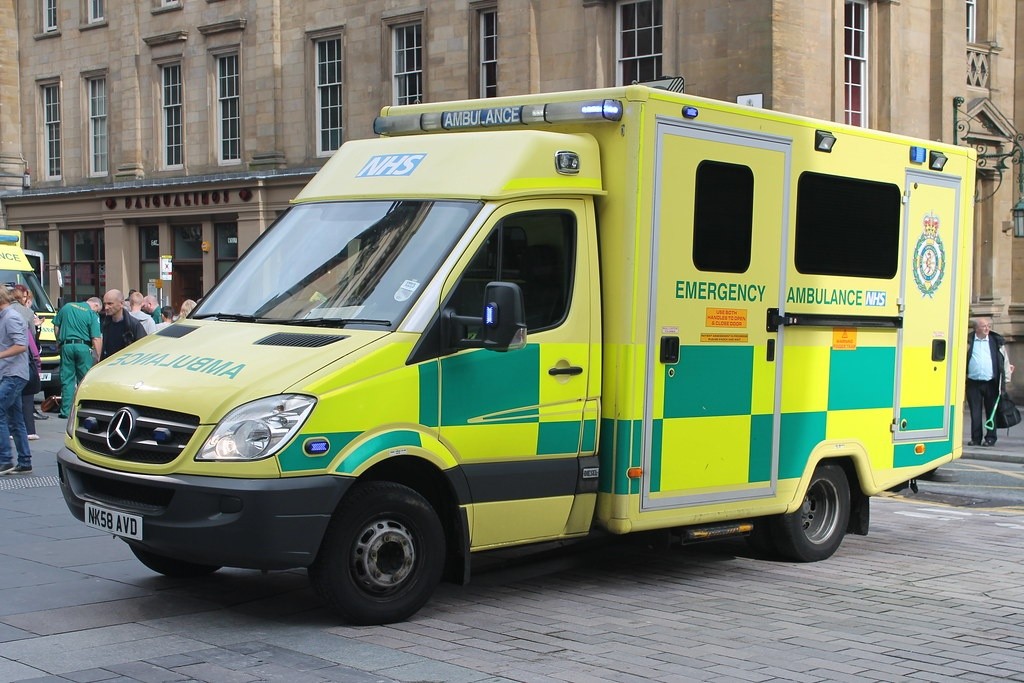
[7,464,32,474]
[0,464,15,475]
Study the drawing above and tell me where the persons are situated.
[0,282,198,476]
[967,318,1011,449]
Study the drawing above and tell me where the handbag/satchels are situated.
[41,395,62,412]
[992,390,1021,429]
[22,355,41,395]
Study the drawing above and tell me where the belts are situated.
[60,339,91,347]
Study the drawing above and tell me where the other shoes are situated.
[9,435,14,439]
[968,439,981,445]
[32,410,49,420]
[983,437,996,446]
[58,414,68,419]
[27,434,39,440]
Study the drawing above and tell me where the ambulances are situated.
[0,232,61,402]
[56,86,977,624]
